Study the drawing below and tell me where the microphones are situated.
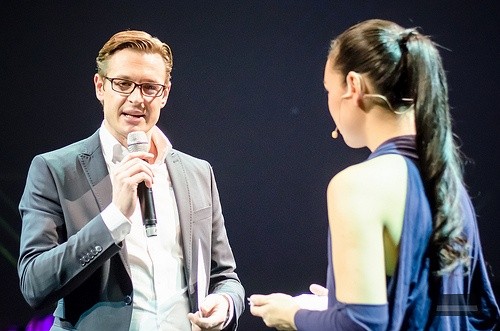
[332,127,338,138]
[128,130,158,237]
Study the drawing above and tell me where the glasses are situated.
[103,75,166,97]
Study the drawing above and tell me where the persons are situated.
[17,30,246,331]
[249,18,499,331]
